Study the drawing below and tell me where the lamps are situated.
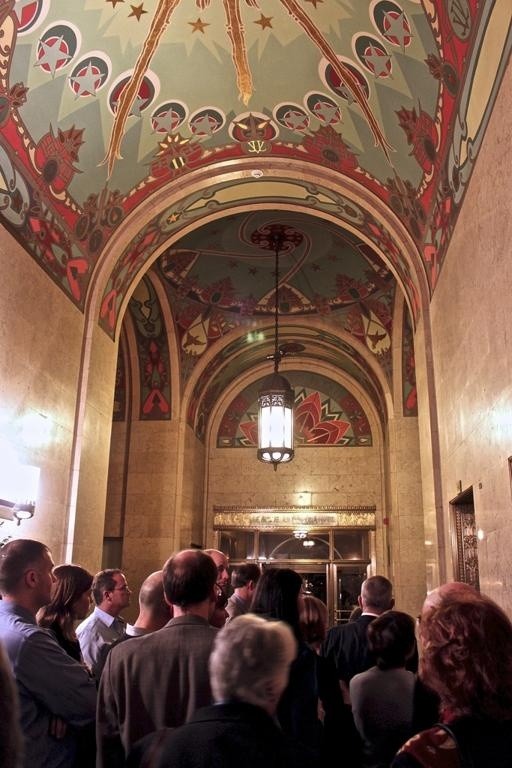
[254,227,297,473]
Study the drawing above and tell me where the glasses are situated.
[107,585,129,592]
